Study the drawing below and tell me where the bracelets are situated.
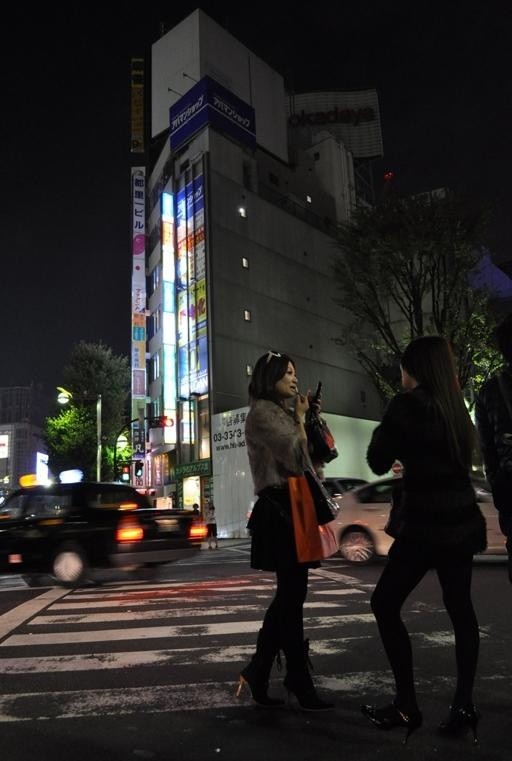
[295,412,305,425]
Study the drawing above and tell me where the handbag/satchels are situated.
[298,450,343,526]
[304,404,340,464]
[286,472,339,565]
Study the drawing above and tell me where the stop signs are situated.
[392,463,401,474]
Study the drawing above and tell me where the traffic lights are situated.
[159,416,173,428]
[135,461,143,477]
[120,465,130,482]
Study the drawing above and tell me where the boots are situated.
[233,626,286,707]
[280,637,337,712]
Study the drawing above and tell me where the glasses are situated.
[265,351,282,363]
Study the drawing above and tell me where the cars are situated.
[318,476,508,565]
[0,482,209,589]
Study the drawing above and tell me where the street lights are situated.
[56,387,102,481]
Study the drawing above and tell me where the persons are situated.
[474,316,512,587]
[364,334,487,737]
[192,504,203,518]
[205,502,220,552]
[235,350,339,714]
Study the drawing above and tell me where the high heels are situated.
[433,701,481,744]
[359,697,425,746]
